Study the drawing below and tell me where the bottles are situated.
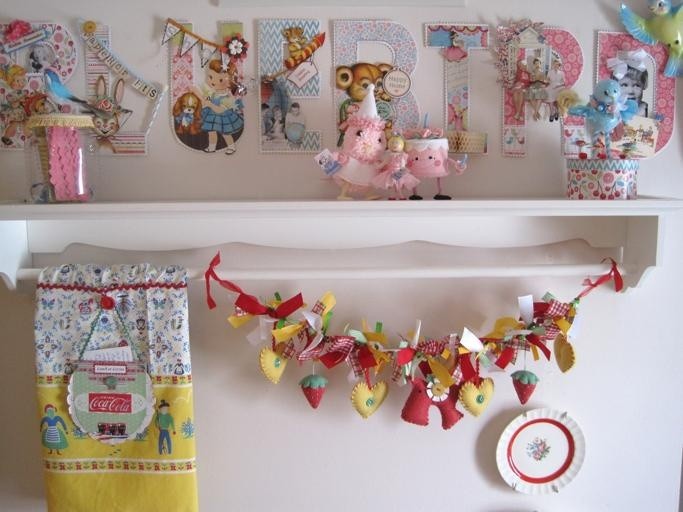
[25,116,97,203]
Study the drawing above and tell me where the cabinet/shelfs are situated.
[1,196,682,292]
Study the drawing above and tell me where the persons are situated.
[0,64,33,145]
[261,103,304,142]
[613,66,650,119]
[200,59,244,155]
[510,58,565,122]
